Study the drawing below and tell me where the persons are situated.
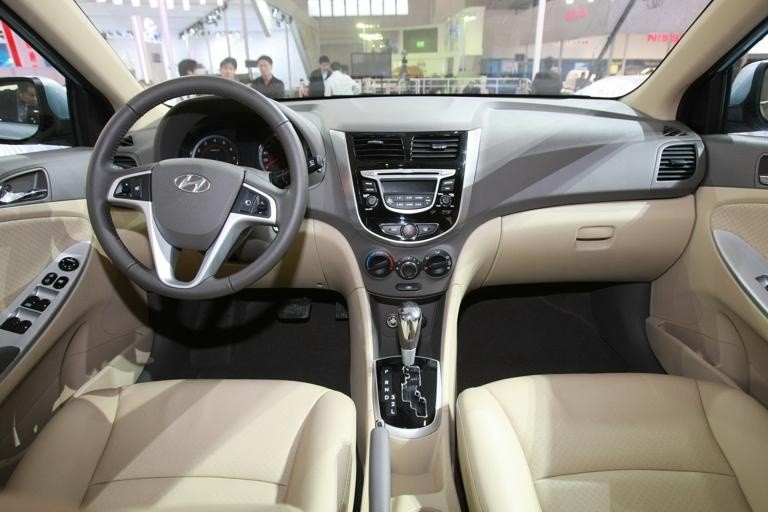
[513,56,588,98]
[322,61,360,98]
[308,55,331,96]
[178,59,199,76]
[217,56,237,80]
[249,55,284,100]
[0,81,39,124]
[461,80,481,95]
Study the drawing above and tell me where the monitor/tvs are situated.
[402,28,438,54]
[350,51,392,78]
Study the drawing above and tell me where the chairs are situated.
[0,377,358,512]
[455,370,768,512]
